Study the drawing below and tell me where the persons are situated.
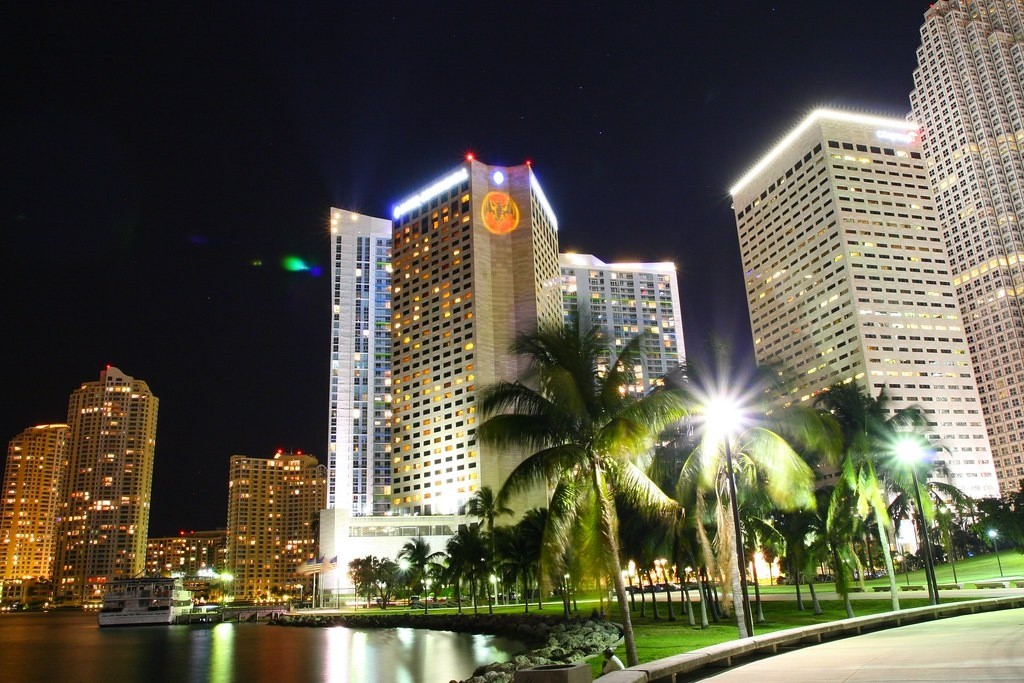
[601,647,625,676]
[589,608,599,620]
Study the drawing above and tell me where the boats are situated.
[95,574,193,624]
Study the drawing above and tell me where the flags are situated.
[296,557,337,576]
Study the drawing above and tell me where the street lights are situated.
[900,439,940,606]
[709,399,754,637]
